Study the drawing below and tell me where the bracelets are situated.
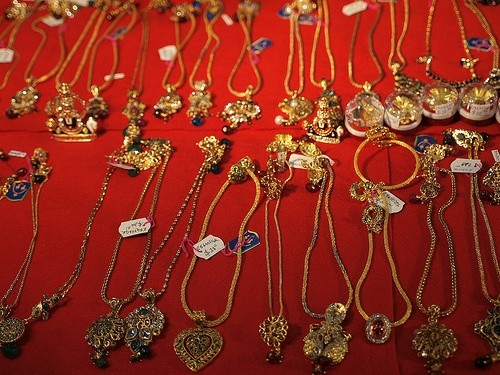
[353,127,421,191]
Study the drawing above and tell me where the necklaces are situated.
[0,0,500,375]
[349,182,413,345]
[260,157,292,364]
[84,138,173,365]
